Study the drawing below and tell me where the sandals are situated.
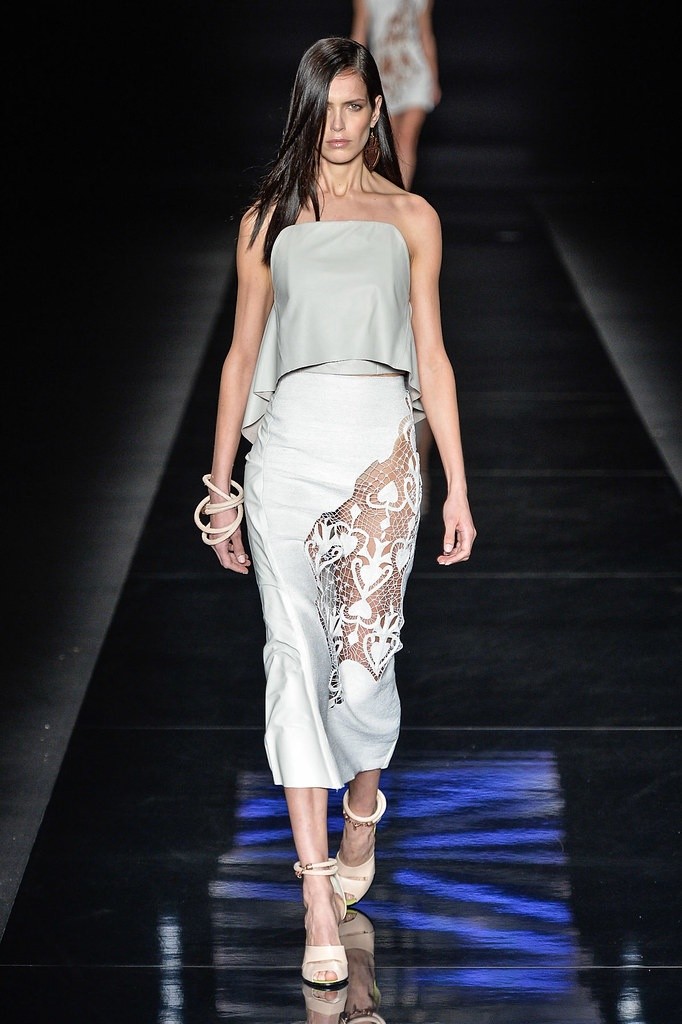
[293,982,349,1024]
[338,910,387,1024]
[294,859,348,992]
[334,786,388,905]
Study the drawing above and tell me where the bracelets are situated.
[195,474,243,545]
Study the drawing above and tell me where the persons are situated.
[303,907,386,1023]
[193,35,476,988]
[352,1,441,193]
